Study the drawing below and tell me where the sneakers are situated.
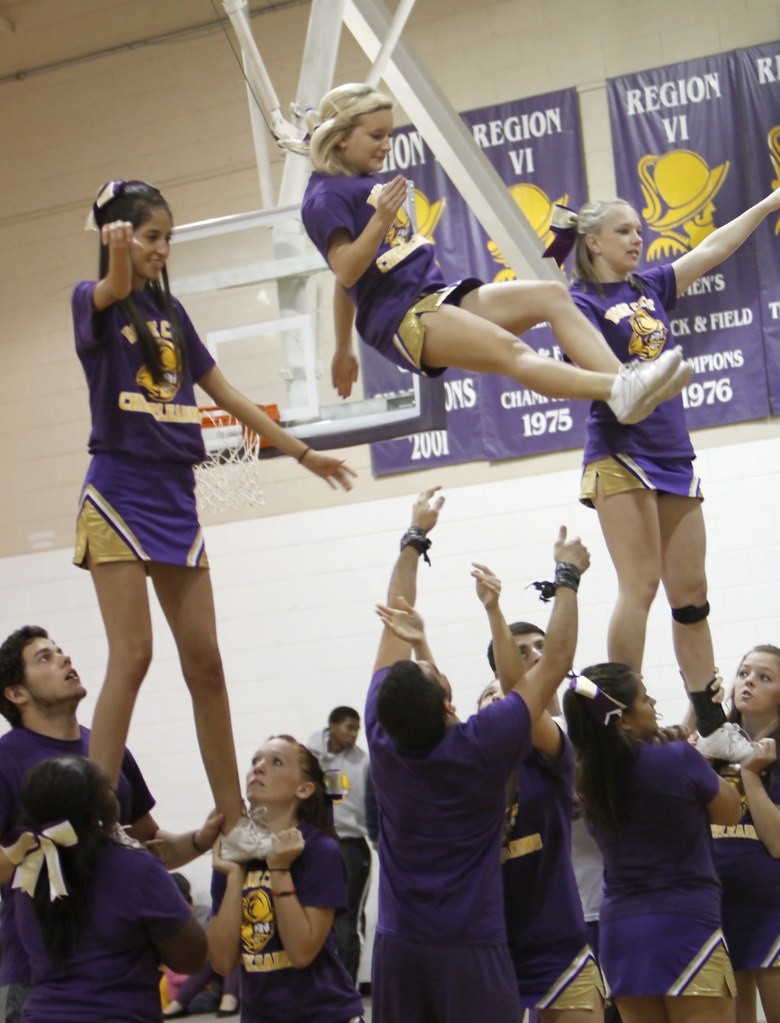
[215,806,277,861]
[694,724,756,762]
[114,823,143,849]
[623,362,693,425]
[606,350,682,422]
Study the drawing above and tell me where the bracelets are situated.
[524,561,581,604]
[400,526,432,569]
[298,445,311,463]
[192,831,205,854]
[269,869,291,872]
[271,887,298,897]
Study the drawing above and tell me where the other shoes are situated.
[216,1001,240,1018]
[162,1008,187,1020]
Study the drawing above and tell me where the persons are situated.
[71,178,359,862]
[562,663,743,1023]
[539,183,778,763]
[304,704,371,1023]
[705,644,779,1023]
[363,482,606,1023]
[210,736,364,1023]
[0,626,248,1023]
[280,82,695,425]
[12,753,210,1023]
